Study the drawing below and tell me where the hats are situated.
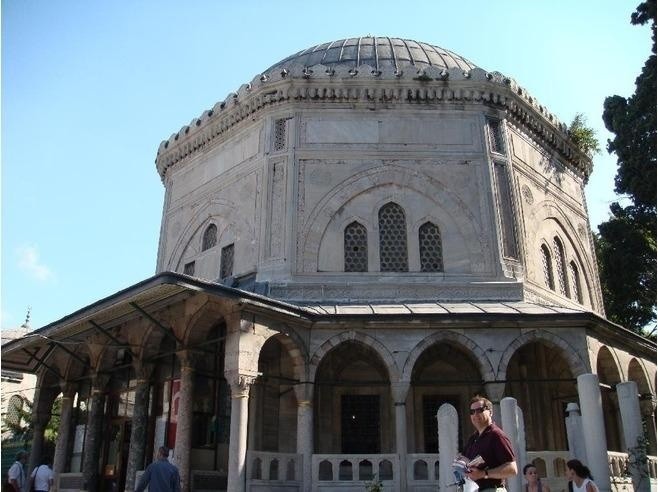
[159,446,169,456]
[15,449,29,460]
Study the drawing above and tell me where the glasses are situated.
[468,406,487,415]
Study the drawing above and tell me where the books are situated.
[452,454,484,476]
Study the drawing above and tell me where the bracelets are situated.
[484,470,488,479]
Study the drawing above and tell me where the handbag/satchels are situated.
[28,481,35,492]
[4,480,16,491]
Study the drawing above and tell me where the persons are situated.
[521,464,551,492]
[7,449,29,491]
[461,396,517,492]
[29,457,53,492]
[134,446,181,492]
[564,459,599,492]
